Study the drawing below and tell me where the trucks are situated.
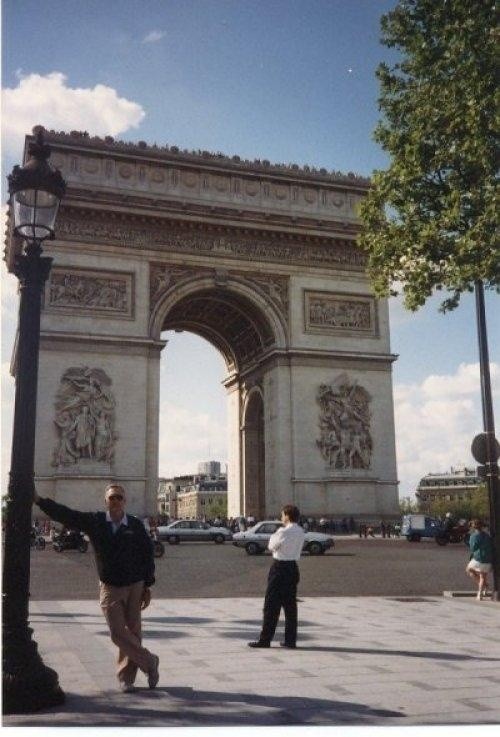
[400,514,450,545]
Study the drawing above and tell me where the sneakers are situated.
[278,638,297,647]
[247,638,271,647]
[115,681,135,693]
[138,652,160,690]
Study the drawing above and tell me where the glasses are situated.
[107,494,123,501]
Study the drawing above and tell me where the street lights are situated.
[2,126,67,714]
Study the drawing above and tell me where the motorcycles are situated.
[52,530,89,553]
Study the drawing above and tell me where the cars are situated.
[154,520,334,556]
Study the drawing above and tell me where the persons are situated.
[143,515,402,538]
[444,512,458,531]
[320,378,371,470]
[248,505,305,648]
[52,379,113,466]
[34,485,159,693]
[466,519,493,601]
